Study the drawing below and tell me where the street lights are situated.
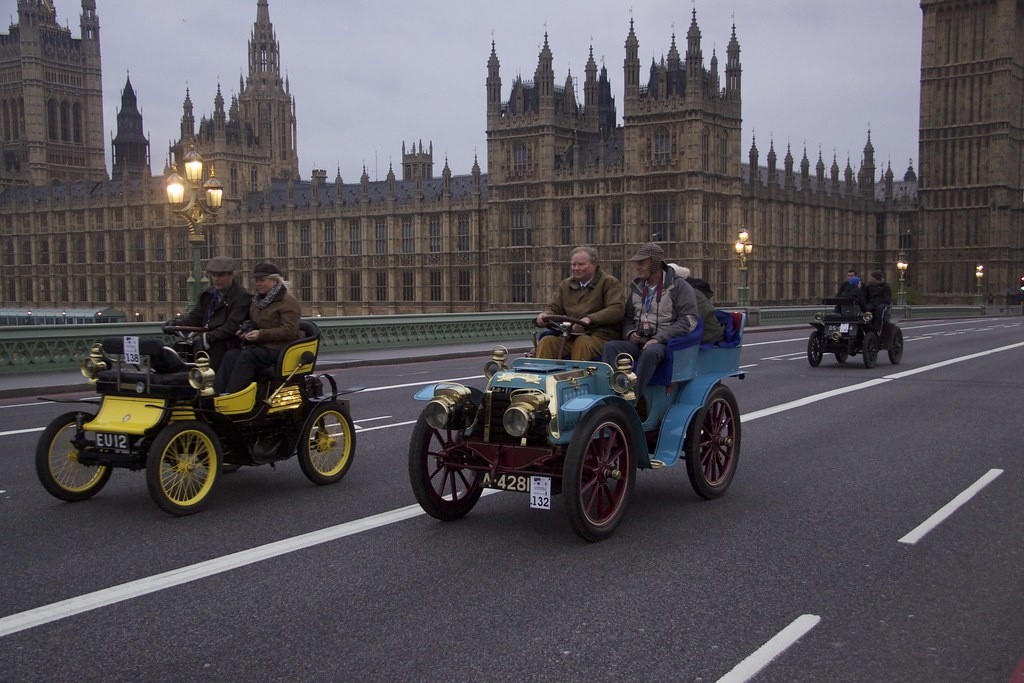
[976,264,986,305]
[733,224,752,308]
[893,247,908,307]
[161,134,223,315]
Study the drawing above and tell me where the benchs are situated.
[700,306,746,374]
[818,297,892,335]
[258,320,322,384]
[537,313,700,385]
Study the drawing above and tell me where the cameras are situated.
[239,320,258,335]
[636,329,656,349]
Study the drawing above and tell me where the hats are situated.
[248,263,281,277]
[205,256,235,272]
[871,270,882,281]
[630,244,664,261]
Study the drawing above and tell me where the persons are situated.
[865,271,891,332]
[535,247,625,361]
[215,263,305,396]
[162,257,248,373]
[841,278,866,314]
[602,243,697,405]
[836,270,856,298]
[666,264,724,342]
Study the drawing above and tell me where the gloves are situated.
[193,333,203,351]
[162,320,182,335]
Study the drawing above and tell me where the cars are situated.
[35,320,353,515]
[806,297,903,369]
[407,312,750,544]
